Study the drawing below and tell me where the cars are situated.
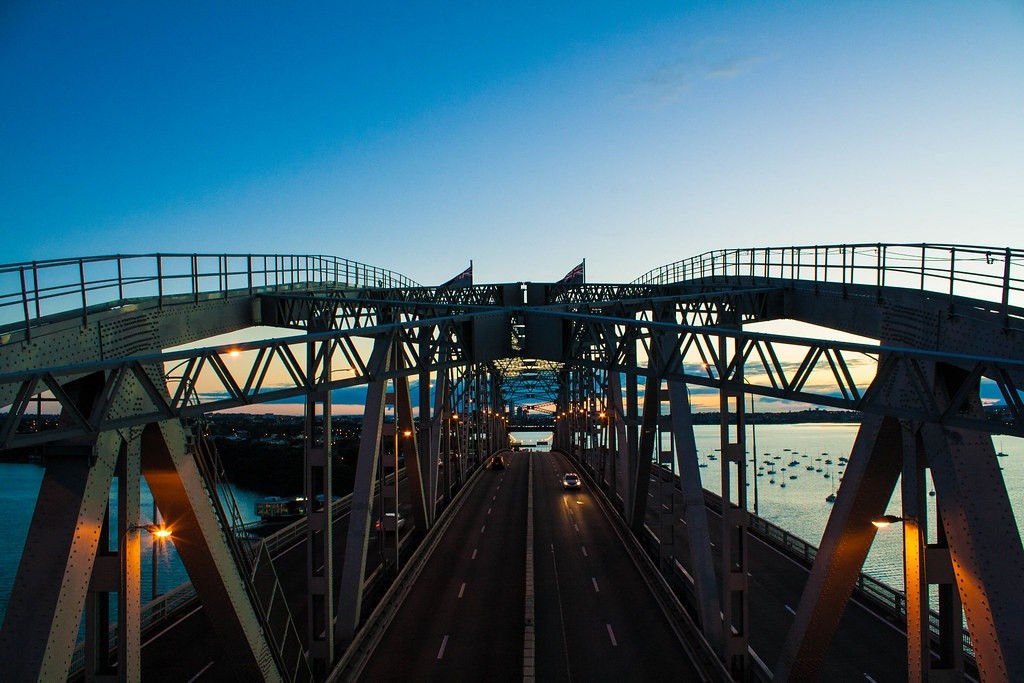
[562,472,582,490]
[375,513,405,533]
[491,457,505,470]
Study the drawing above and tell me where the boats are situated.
[699,449,850,503]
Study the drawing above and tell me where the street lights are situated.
[117,522,173,683]
[869,514,932,683]
[304,368,355,514]
[701,363,759,515]
[152,348,241,600]
[379,430,413,513]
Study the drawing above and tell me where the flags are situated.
[440,266,472,292]
[553,263,584,297]
